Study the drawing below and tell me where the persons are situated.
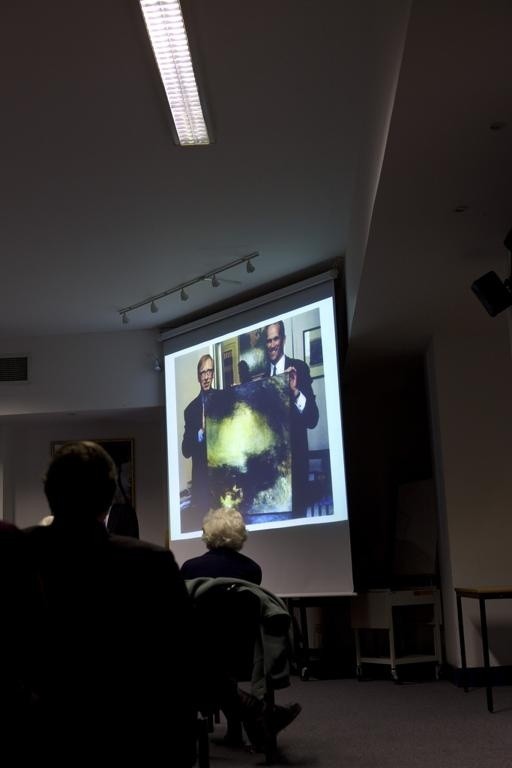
[260,320,320,480]
[180,506,263,750]
[0,438,303,768]
[181,355,225,505]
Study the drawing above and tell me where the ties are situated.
[273,367,276,376]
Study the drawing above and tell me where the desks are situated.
[455,586,512,712]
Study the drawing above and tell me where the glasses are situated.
[199,369,213,378]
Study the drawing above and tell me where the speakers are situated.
[470,270,512,319]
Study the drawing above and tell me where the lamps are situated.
[134,0,215,149]
[119,250,259,324]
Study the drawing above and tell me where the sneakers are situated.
[243,702,301,753]
